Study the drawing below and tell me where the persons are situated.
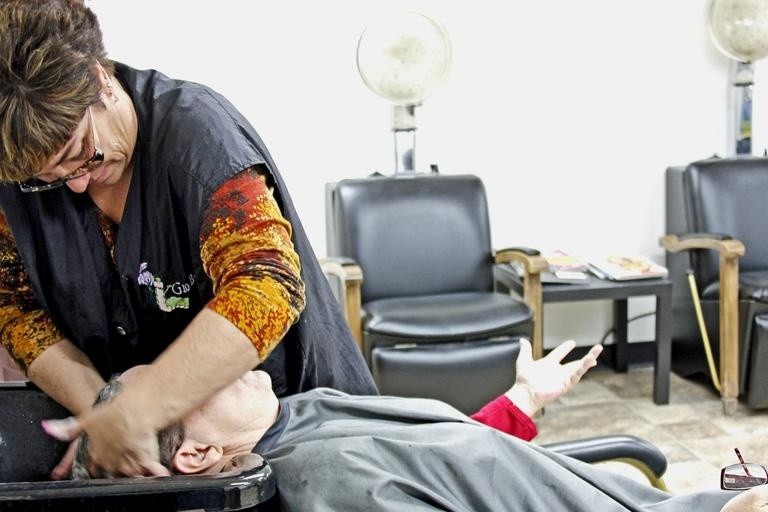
[80,340,768,512]
[0,0,381,481]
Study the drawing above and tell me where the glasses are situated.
[719,449,768,492]
[19,106,105,194]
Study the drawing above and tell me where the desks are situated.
[491,264,673,405]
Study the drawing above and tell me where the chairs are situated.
[319,174,550,420]
[660,158,766,413]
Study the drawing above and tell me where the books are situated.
[511,248,669,283]
